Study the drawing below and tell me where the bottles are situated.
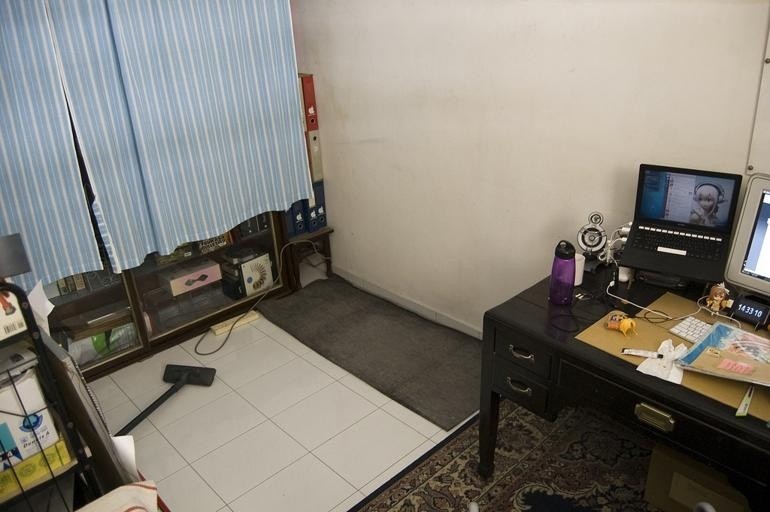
[549,239,576,305]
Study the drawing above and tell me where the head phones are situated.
[692,181,726,203]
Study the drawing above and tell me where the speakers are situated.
[576,209,607,255]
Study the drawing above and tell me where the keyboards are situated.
[669,316,712,344]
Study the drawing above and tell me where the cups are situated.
[574,254,586,287]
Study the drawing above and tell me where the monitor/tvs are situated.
[723,172,770,298]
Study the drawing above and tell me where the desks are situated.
[483,262,768,511]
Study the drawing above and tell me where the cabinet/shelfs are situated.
[0,210,292,384]
[0,278,107,511]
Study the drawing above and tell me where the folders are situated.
[278,71,327,240]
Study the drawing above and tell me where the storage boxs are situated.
[642,442,749,512]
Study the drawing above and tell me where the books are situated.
[673,320,770,387]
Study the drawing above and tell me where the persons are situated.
[706,284,729,315]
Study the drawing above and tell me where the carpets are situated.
[343,396,647,511]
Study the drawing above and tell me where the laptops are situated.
[618,162,743,285]
